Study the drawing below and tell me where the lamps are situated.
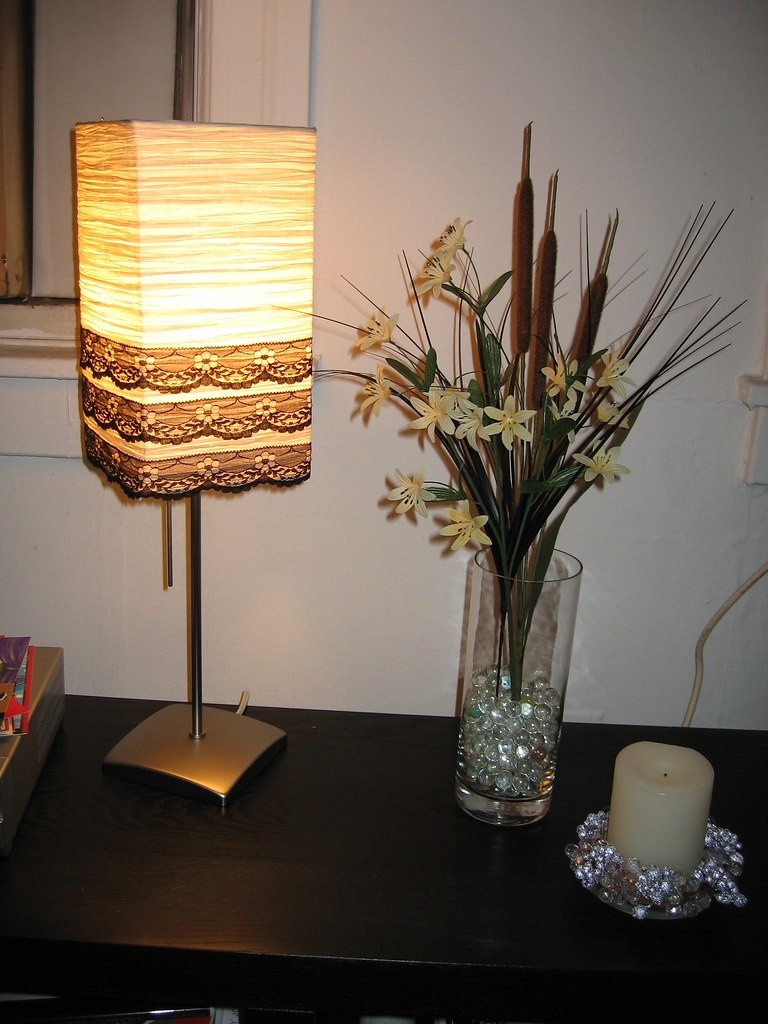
[72,119,317,806]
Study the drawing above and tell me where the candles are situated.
[607,740,714,874]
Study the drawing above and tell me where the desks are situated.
[0,694,768,1024]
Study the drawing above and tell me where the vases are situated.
[455,549,583,825]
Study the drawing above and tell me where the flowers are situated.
[269,119,746,709]
